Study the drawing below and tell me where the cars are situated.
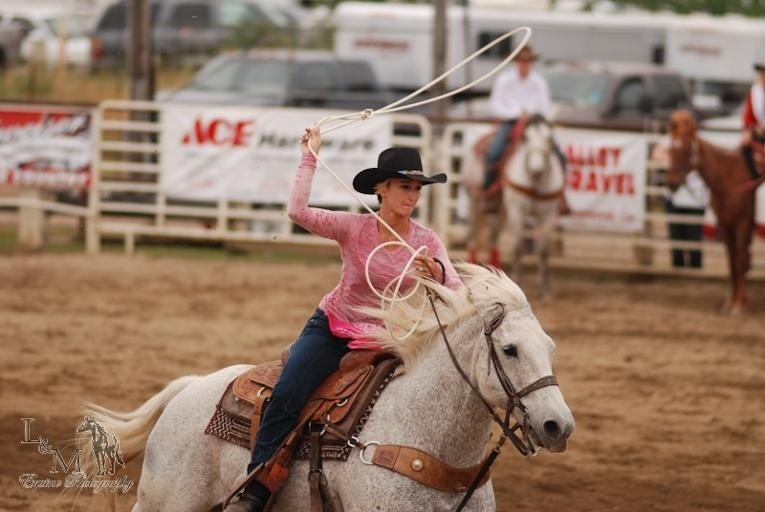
[155,48,396,111]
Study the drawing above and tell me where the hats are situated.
[509,45,539,62]
[352,147,447,194]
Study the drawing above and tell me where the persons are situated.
[658,145,707,270]
[741,60,765,184]
[482,46,551,191]
[219,124,472,512]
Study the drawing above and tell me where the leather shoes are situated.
[225,477,276,512]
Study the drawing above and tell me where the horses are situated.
[74,258,577,511]
[457,104,566,305]
[664,122,765,321]
